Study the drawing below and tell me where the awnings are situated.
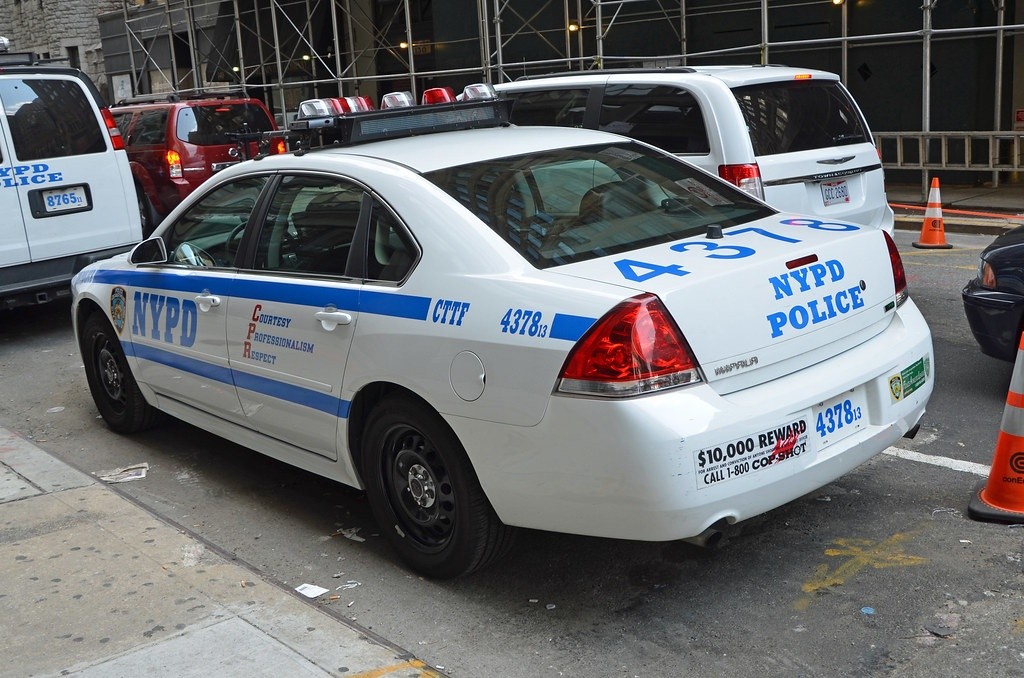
[205,0,347,83]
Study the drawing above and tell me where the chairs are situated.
[287,191,362,275]
[578,181,652,224]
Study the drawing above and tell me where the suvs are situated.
[106,96,287,240]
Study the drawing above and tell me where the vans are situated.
[0,36,143,326]
[458,63,894,249]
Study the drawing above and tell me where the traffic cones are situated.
[967,328,1024,523]
[911,176,953,250]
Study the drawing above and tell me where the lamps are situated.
[569,25,580,32]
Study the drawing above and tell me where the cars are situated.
[70,82,934,583]
[961,223,1024,364]
